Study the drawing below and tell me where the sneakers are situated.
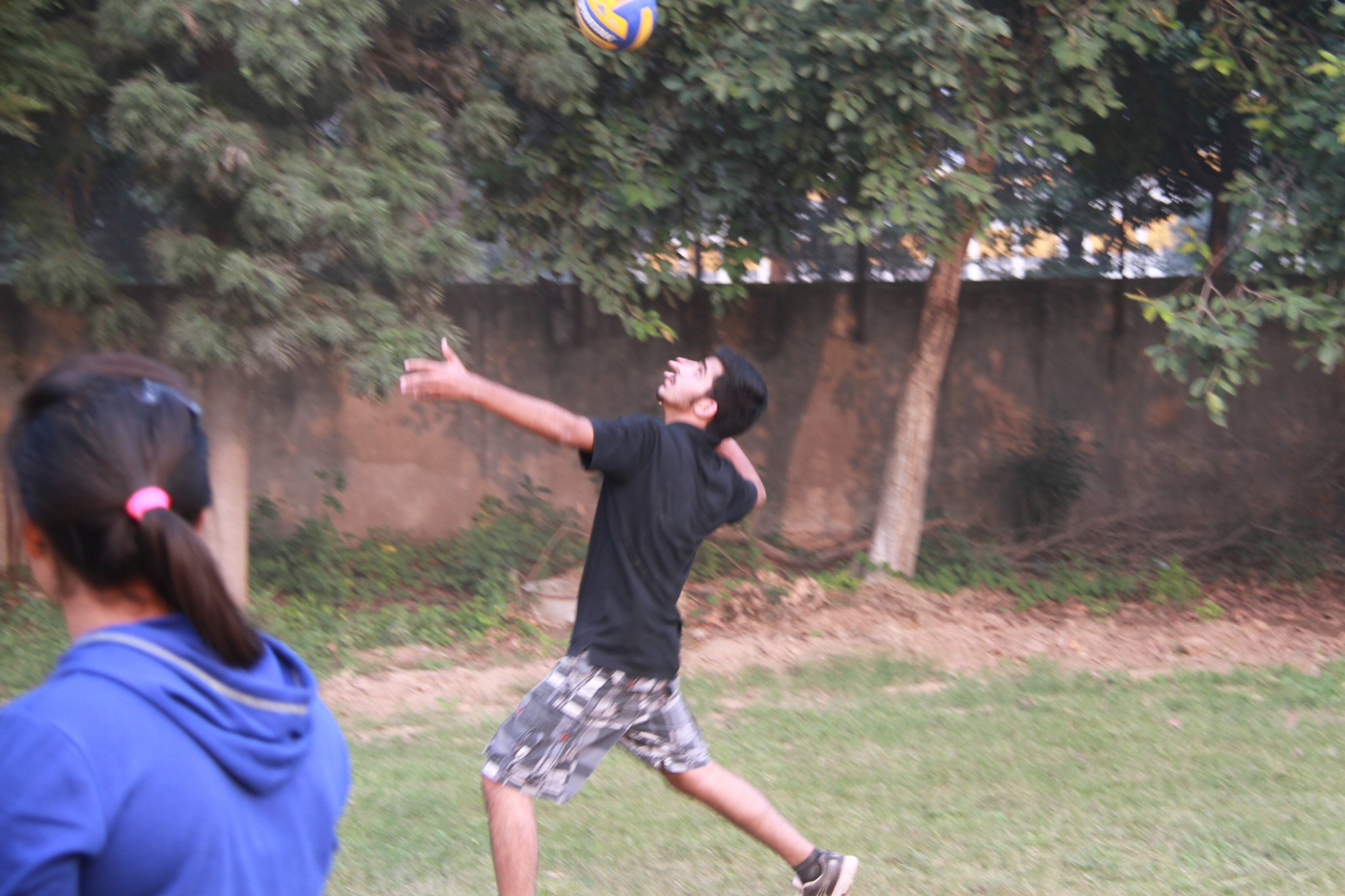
[791,854,859,896]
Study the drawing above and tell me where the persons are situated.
[1,355,353,894]
[399,338,862,896]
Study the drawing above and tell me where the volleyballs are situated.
[575,0,659,53]
